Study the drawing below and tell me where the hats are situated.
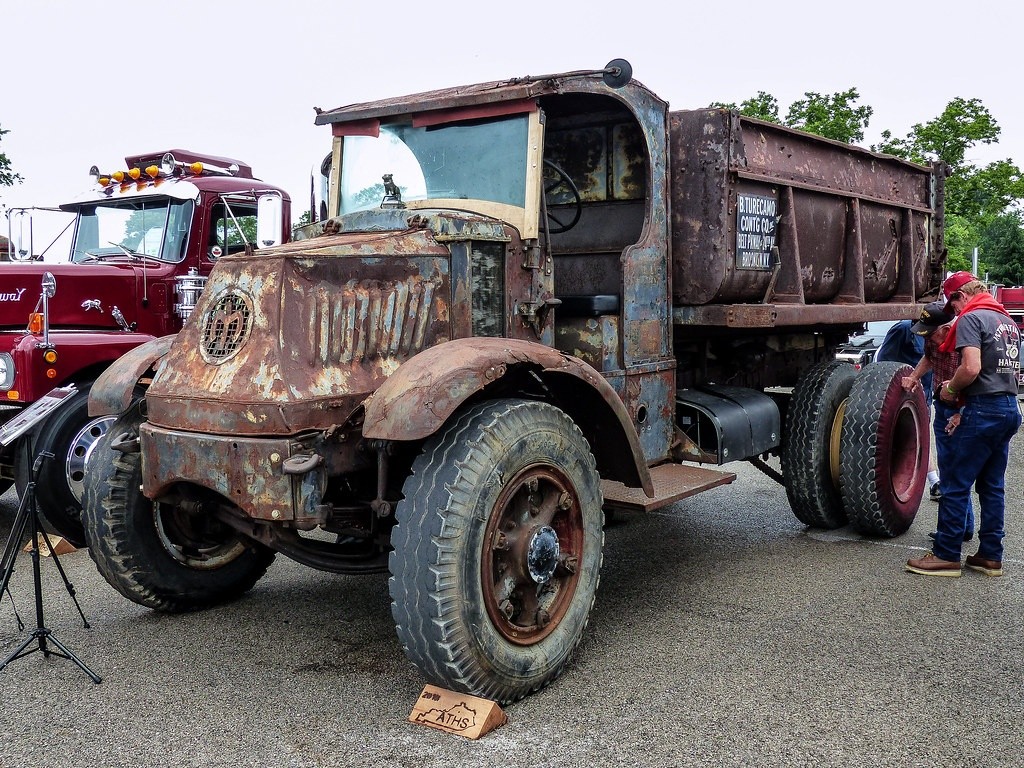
[910,301,955,336]
[942,271,975,315]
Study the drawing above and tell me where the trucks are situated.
[837,248,1024,366]
[0,146,332,548]
[82,62,947,703]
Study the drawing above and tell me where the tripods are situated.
[0,387,102,685]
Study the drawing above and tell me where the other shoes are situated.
[930,481,941,499]
[928,531,968,542]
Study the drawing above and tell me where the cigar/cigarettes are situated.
[950,421,957,429]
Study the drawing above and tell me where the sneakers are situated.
[966,554,1003,576]
[905,554,961,577]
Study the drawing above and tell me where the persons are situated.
[906,271,1022,577]
[901,302,973,542]
[872,318,942,500]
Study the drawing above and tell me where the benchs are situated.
[219,243,256,254]
[554,291,622,314]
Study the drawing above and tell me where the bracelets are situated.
[947,382,955,394]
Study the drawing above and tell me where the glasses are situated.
[923,325,951,340]
